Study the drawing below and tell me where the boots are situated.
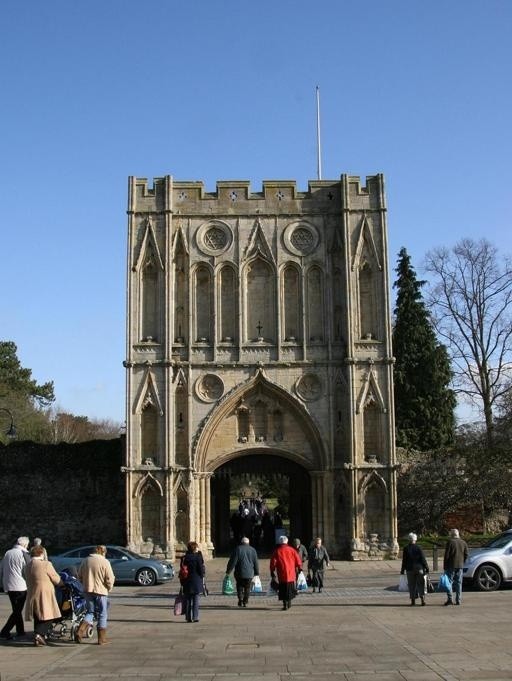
[75,620,89,644]
[97,627,112,645]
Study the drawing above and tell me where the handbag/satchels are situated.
[297,570,308,589]
[306,568,312,587]
[267,575,279,595]
[250,575,262,592]
[271,578,279,592]
[437,571,454,593]
[180,557,188,577]
[174,585,186,616]
[423,574,428,594]
[427,574,435,593]
[223,574,234,595]
[398,574,409,592]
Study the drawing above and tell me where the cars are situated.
[47,544,174,587]
[462,529,512,591]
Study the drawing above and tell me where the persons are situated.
[29,538,48,561]
[262,513,275,553]
[226,537,259,607]
[179,542,205,621]
[400,533,429,605]
[310,541,315,549]
[291,538,307,573]
[270,536,303,609]
[443,529,469,605]
[74,545,115,645]
[308,538,330,592]
[0,536,29,640]
[21,546,64,647]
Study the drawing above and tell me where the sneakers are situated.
[0,634,16,641]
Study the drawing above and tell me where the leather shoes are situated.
[457,600,460,605]
[445,600,453,606]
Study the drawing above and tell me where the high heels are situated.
[35,634,47,646]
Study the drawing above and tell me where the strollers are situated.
[49,569,109,644]
